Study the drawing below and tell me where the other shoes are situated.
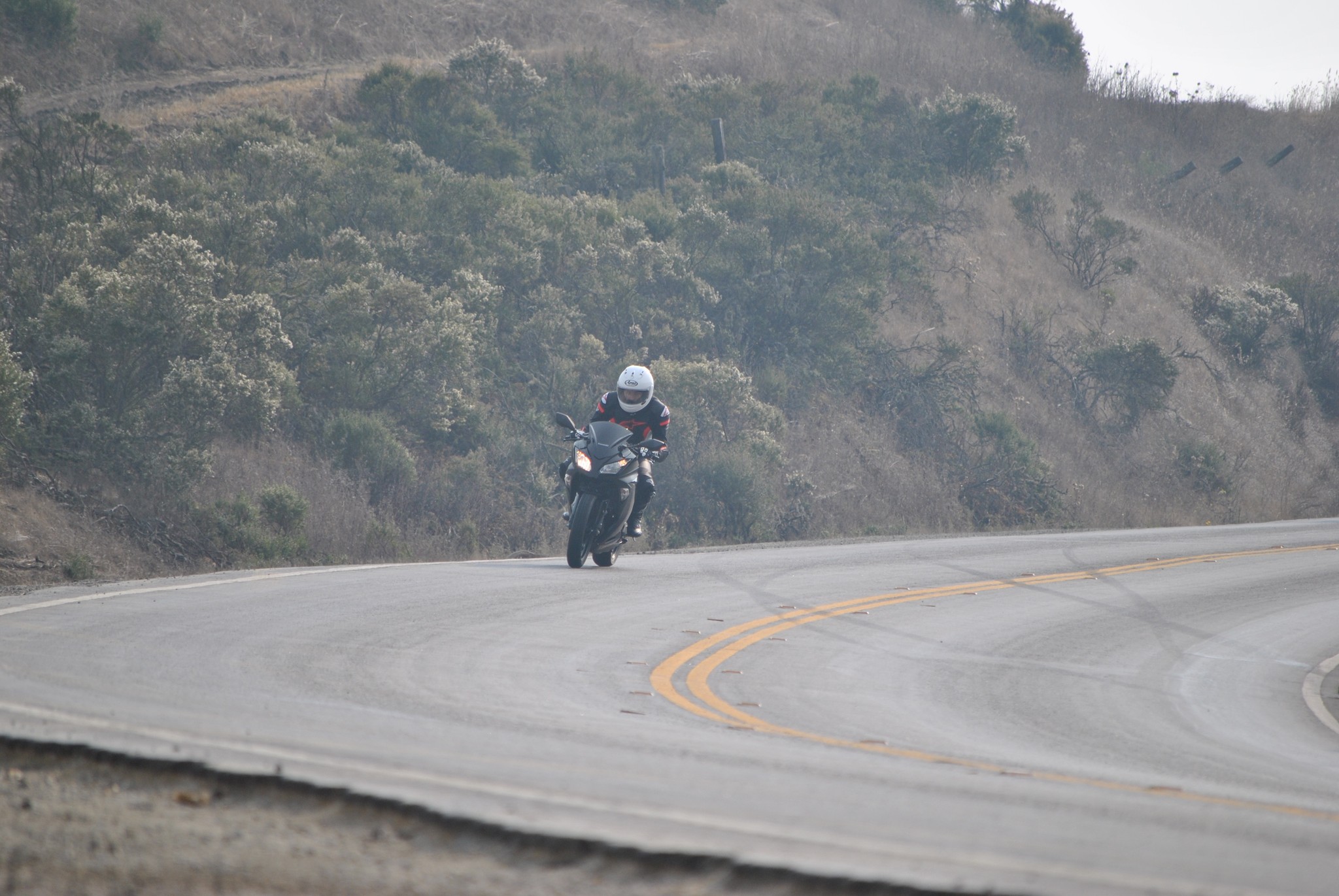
[627,512,642,536]
[562,512,570,521]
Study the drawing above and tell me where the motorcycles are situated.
[555,411,666,569]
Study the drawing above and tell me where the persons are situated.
[558,365,670,536]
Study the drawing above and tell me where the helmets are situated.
[617,365,654,413]
